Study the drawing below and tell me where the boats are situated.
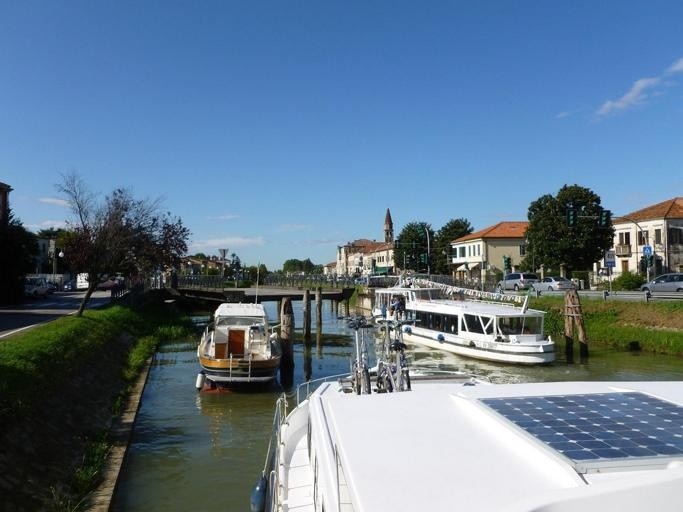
[355,283,555,365]
[249,302,683,512]
[196,302,283,384]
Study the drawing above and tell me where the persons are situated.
[381,295,412,323]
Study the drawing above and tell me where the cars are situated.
[497,273,540,292]
[45,280,56,292]
[63,279,76,292]
[76,272,124,289]
[639,273,682,292]
[23,277,48,299]
[530,276,577,291]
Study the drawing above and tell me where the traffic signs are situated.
[604,250,614,266]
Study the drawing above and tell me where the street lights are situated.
[52,248,64,283]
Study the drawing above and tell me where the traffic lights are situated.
[565,209,577,231]
[598,210,609,227]
[504,257,511,269]
